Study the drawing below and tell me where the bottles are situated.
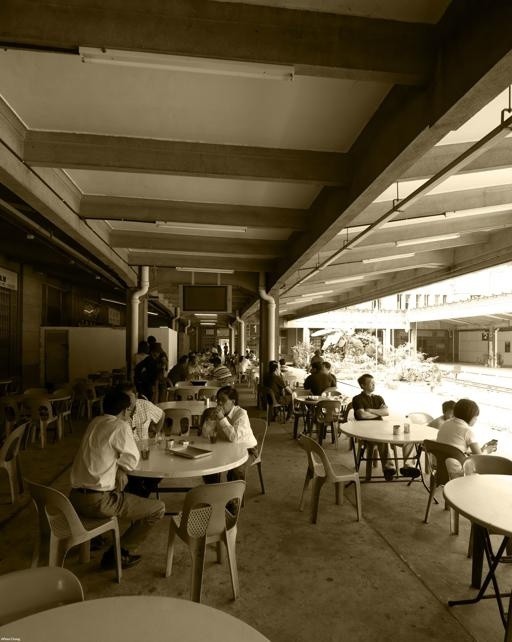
[404,415,411,434]
[167,438,175,448]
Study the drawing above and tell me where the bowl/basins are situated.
[190,380,206,386]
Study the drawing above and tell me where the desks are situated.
[339,421,440,507]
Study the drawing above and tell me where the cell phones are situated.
[488,439,498,446]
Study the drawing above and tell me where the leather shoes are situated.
[98,548,143,572]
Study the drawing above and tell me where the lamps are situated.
[176,267,235,274]
[78,46,294,82]
[156,220,247,233]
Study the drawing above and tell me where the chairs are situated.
[240,374,264,397]
[244,418,267,494]
[295,432,362,524]
[165,479,247,603]
[0,371,237,506]
[464,454,512,558]
[258,375,356,449]
[423,439,466,534]
[348,409,379,467]
[405,412,433,473]
[19,477,122,582]
[0,568,84,625]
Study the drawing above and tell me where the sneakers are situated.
[383,468,396,481]
[400,467,421,478]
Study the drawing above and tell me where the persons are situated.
[115,383,165,441]
[430,398,497,475]
[133,335,337,423]
[202,386,258,515]
[352,374,396,475]
[69,391,166,570]
[427,400,456,429]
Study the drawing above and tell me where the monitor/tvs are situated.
[178,284,233,314]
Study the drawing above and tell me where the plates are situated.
[165,445,213,460]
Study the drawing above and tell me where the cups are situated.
[154,432,166,451]
[208,431,217,444]
[326,391,331,398]
[296,382,299,387]
[392,424,401,435]
[138,438,151,460]
